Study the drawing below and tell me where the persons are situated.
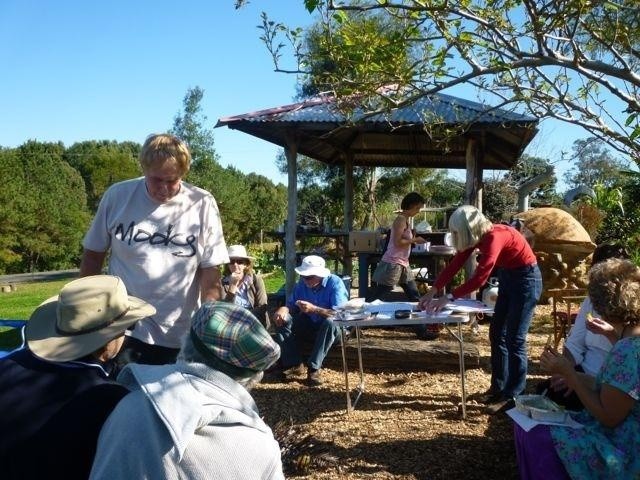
[274,255,348,385]
[348,192,440,340]
[514,259,640,479]
[536,244,631,411]
[222,245,271,329]
[417,205,542,416]
[79,134,230,366]
[0,274,157,480]
[88,301,283,480]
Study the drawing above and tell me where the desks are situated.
[334,305,470,421]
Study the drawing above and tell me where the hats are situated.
[25,274,157,364]
[227,244,253,259]
[189,300,281,379]
[294,255,330,278]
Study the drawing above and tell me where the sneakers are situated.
[487,397,511,414]
[307,369,324,384]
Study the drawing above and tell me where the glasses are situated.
[230,258,244,264]
